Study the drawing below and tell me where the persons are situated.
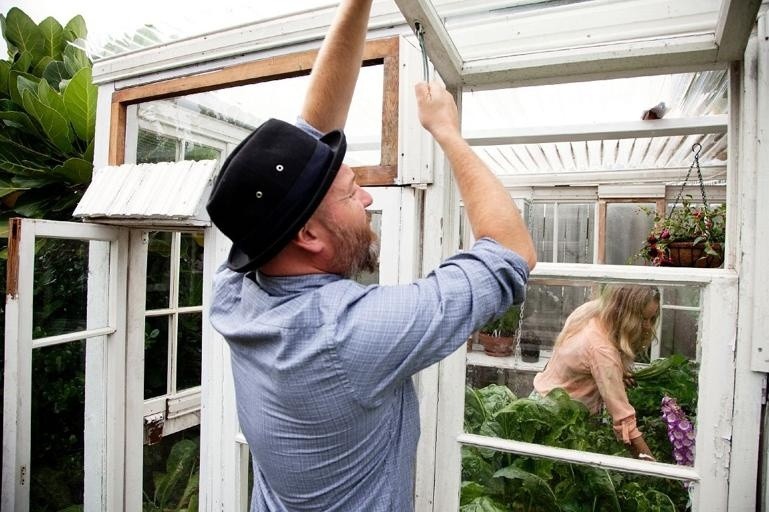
[527,281,666,465]
[206,0,540,511]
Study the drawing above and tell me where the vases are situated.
[659,242,725,268]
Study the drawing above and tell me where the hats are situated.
[202,113,350,276]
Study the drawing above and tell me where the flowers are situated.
[629,195,727,267]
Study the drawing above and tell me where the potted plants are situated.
[477,308,521,358]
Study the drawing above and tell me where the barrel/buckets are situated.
[518,336,542,363]
